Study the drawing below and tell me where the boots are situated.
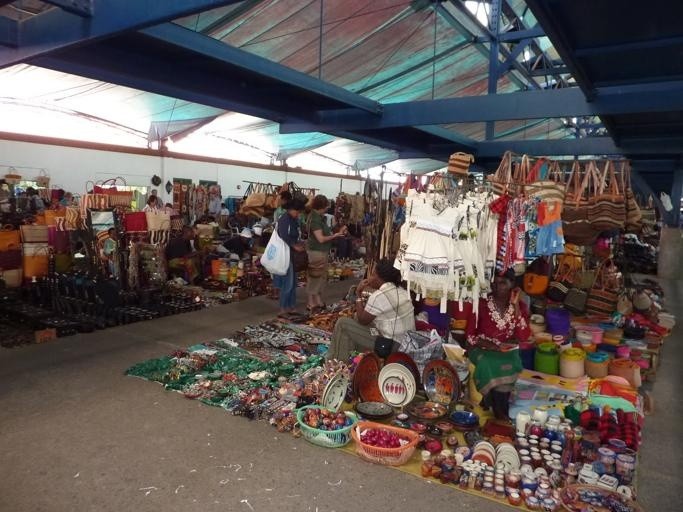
[491,389,511,421]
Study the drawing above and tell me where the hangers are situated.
[412,174,559,211]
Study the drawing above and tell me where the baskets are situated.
[351,421,420,466]
[297,405,358,448]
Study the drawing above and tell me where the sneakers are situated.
[306,302,327,311]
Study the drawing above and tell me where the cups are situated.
[483,466,503,497]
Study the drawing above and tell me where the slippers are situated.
[277,311,304,319]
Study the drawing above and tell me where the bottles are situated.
[508,407,635,512]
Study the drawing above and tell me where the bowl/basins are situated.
[405,402,447,424]
[390,421,444,440]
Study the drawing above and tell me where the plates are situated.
[381,376,407,405]
[355,401,394,421]
[421,361,460,404]
[449,411,480,428]
[378,363,416,408]
[471,441,520,472]
[322,373,347,411]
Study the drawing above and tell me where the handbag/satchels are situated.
[483,180,657,246]
[448,154,471,177]
[625,326,645,340]
[81,185,133,219]
[0,206,92,289]
[239,191,315,219]
[398,330,447,384]
[307,263,324,278]
[374,336,394,358]
[125,212,147,231]
[5,175,20,184]
[260,229,290,276]
[36,176,49,187]
[523,272,652,315]
[544,309,570,335]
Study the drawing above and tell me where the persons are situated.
[328,259,419,364]
[463,266,531,426]
[1,186,405,320]
